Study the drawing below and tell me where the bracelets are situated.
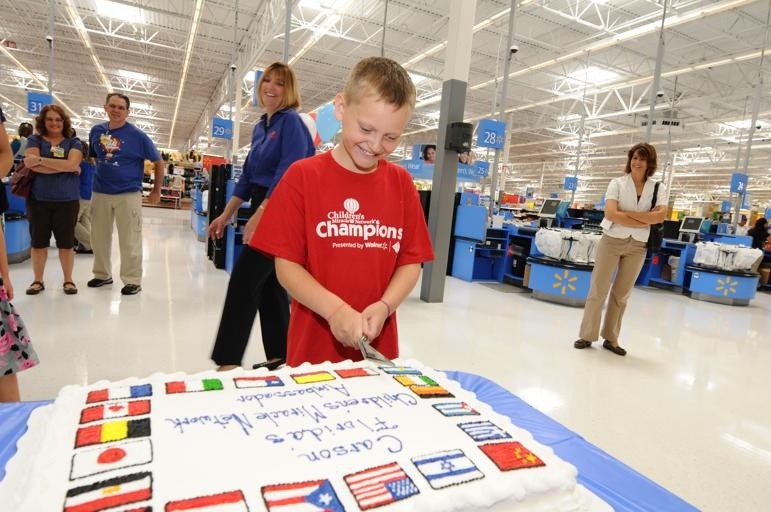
[380,298,391,319]
[257,204,266,211]
[325,301,346,321]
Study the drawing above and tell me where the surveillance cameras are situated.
[230,64,237,71]
[657,91,665,97]
[756,124,761,129]
[510,45,519,53]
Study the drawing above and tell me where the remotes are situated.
[46,36,53,42]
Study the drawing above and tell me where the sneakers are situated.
[121,284,142,294]
[88,278,114,287]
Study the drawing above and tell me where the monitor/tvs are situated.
[538,197,562,229]
[679,215,705,242]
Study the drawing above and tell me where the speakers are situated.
[445,122,473,153]
[207,163,226,260]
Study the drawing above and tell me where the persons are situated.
[746,218,771,252]
[421,144,436,164]
[0,93,165,296]
[458,150,472,165]
[207,61,318,372]
[0,216,42,404]
[247,56,437,369]
[734,215,749,236]
[574,142,669,358]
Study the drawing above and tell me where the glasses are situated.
[45,118,64,123]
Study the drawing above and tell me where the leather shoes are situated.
[63,282,78,293]
[603,340,626,356]
[26,281,45,295]
[574,338,592,349]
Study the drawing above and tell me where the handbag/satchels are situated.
[647,223,664,252]
[11,161,32,198]
